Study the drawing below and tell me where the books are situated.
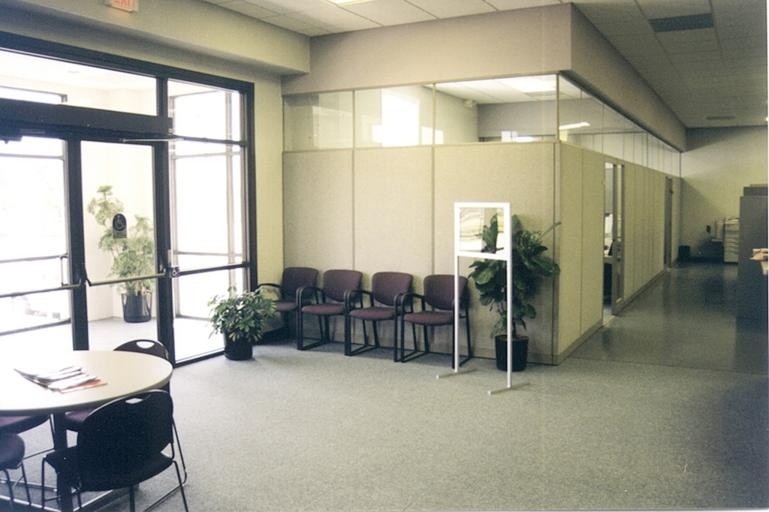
[13,359,107,394]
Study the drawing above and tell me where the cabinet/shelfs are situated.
[734,195,768,321]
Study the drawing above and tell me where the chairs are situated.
[347,271,417,362]
[0,338,189,512]
[400,274,472,369]
[299,269,368,355]
[254,267,323,349]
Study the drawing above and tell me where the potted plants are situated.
[207,284,278,360]
[466,211,561,372]
[86,183,155,323]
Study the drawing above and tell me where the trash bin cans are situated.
[679,246,690,262]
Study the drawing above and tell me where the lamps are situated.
[0,86,68,105]
